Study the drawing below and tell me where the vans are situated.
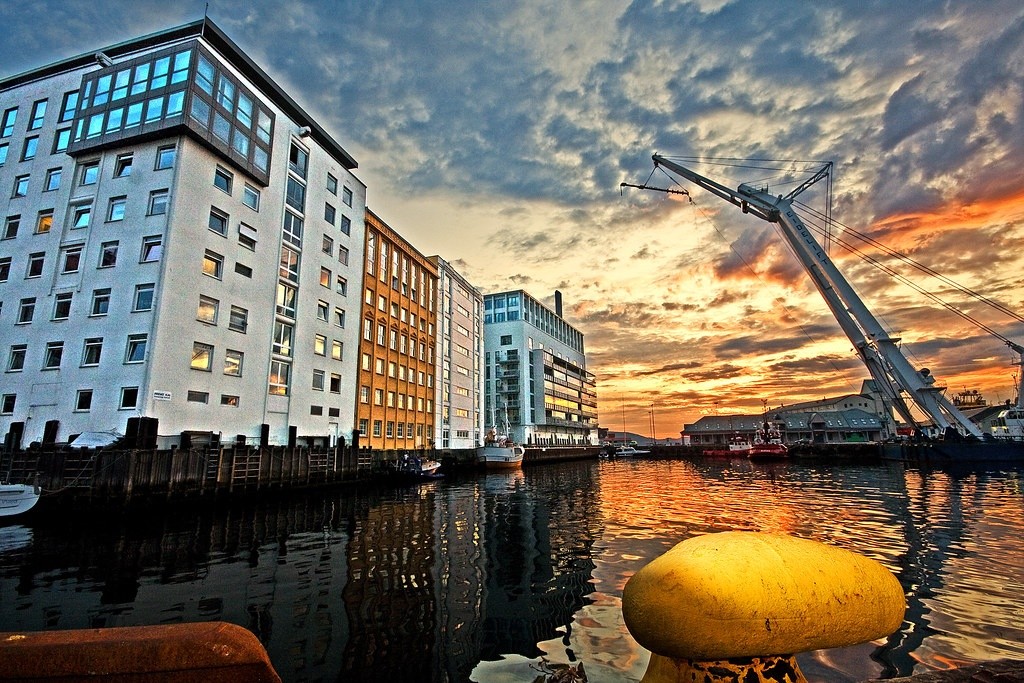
[891,435,909,442]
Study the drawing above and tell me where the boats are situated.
[408,474,445,499]
[475,402,525,468]
[0,521,35,552]
[701,408,788,460]
[599,445,651,458]
[485,468,525,528]
[401,456,441,476]
[0,473,42,516]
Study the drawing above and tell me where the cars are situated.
[795,438,813,445]
[627,441,637,446]
[67,430,124,448]
